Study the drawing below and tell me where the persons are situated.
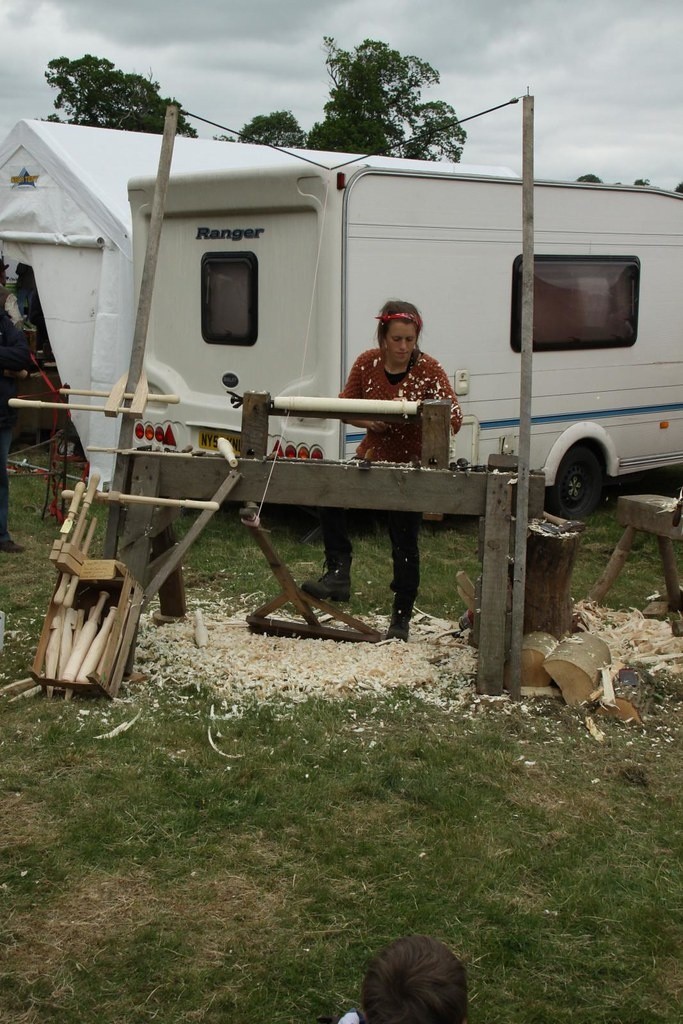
[302,298,464,644]
[315,932,470,1024]
[0,256,49,554]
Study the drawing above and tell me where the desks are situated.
[14,373,67,447]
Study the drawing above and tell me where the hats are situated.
[0,258,9,272]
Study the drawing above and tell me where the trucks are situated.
[126,157,683,522]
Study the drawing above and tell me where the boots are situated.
[302,549,350,602]
[385,594,412,644]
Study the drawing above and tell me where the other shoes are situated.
[60,454,87,463]
[0,540,26,553]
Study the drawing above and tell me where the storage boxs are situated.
[27,559,144,700]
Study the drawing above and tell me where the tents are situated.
[0,117,523,494]
